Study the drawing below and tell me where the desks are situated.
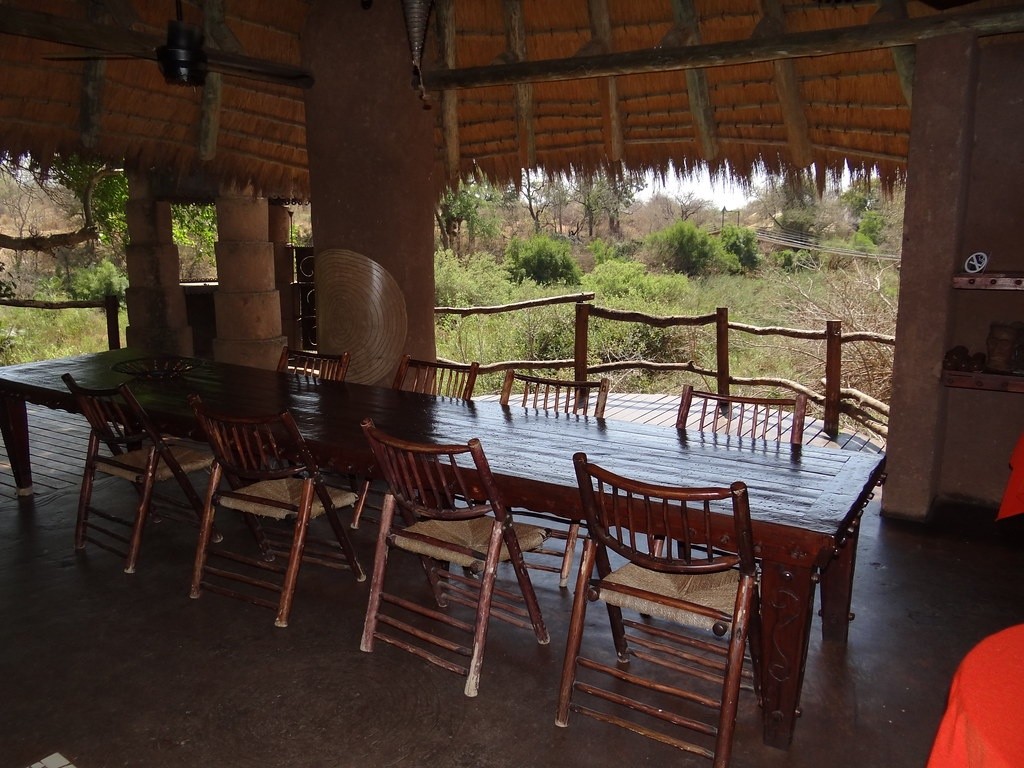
[0,349,887,748]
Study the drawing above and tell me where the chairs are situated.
[62,373,222,573]
[555,452,762,768]
[272,346,806,638]
[187,393,368,630]
[360,417,551,697]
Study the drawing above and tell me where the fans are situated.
[42,1,316,90]
